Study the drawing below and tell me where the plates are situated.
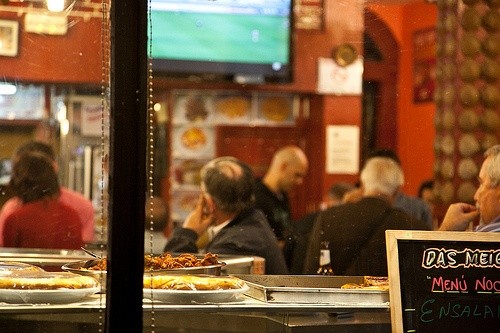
[0,289,101,304]
[143,284,249,303]
[61,261,227,293]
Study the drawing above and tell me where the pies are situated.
[0,273,98,291]
[341,275,389,292]
[142,275,246,291]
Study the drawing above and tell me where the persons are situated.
[3,154,84,249]
[164,154,287,277]
[437,144,500,234]
[254,144,308,270]
[288,148,433,276]
[0,141,94,250]
[144,198,167,254]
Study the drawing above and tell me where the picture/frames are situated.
[0,20,18,57]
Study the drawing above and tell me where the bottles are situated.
[316,242,334,276]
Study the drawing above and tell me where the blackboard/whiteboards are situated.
[385,229,500,333]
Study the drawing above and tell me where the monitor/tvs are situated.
[148,0,294,83]
